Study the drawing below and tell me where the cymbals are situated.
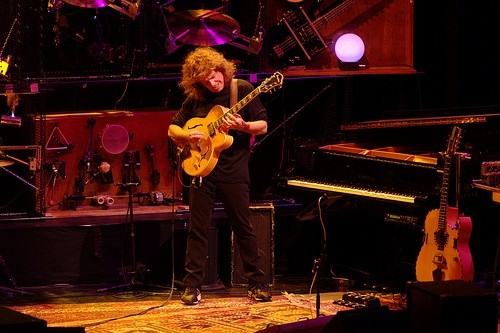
[163,9,241,47]
[62,0,115,9]
[97,124,130,154]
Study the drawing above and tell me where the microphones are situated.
[91,162,111,180]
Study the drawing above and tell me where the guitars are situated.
[415,126,473,281]
[178,71,284,178]
[273,0,385,60]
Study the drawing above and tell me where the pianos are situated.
[283,113,500,309]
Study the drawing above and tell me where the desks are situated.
[0,196,301,287]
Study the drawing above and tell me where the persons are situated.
[167,47,272,306]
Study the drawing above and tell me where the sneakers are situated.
[181,286,202,304]
[247,284,273,301]
